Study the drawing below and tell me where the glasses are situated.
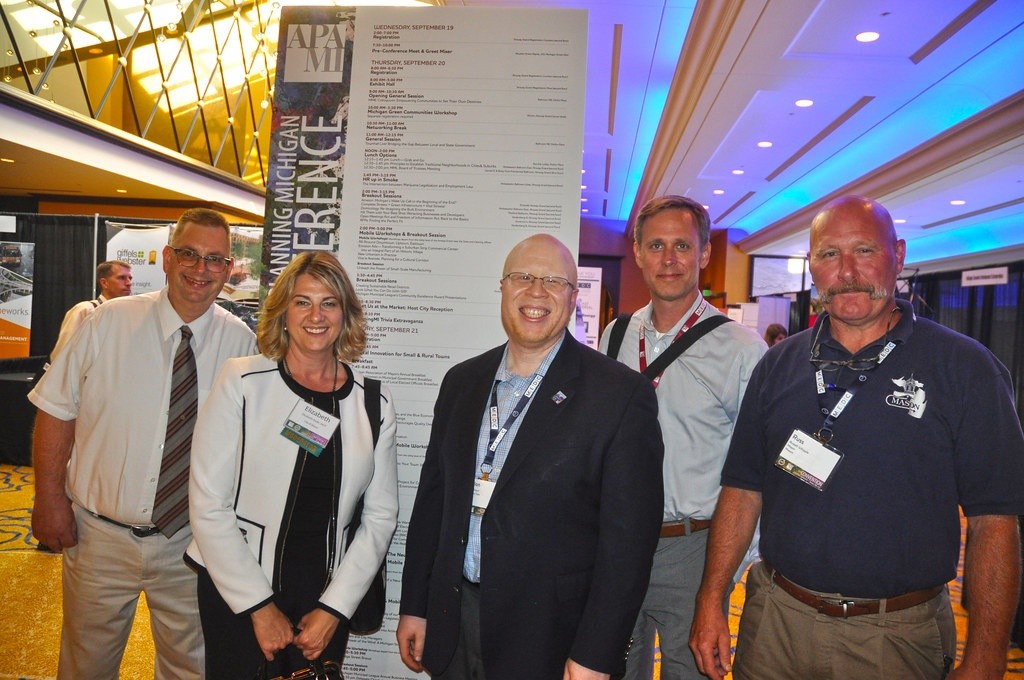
[809,308,901,371]
[174,248,232,273]
[503,272,574,292]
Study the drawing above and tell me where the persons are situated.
[689,196,1024,680]
[184,251,397,679]
[51,260,132,362]
[598,196,769,680]
[397,235,663,680]
[764,324,787,348]
[27,209,258,680]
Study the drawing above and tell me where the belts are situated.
[763,562,944,618]
[88,511,161,538]
[659,517,713,538]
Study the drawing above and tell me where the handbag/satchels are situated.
[252,629,347,680]
[351,378,389,636]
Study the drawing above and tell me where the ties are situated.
[151,327,198,539]
